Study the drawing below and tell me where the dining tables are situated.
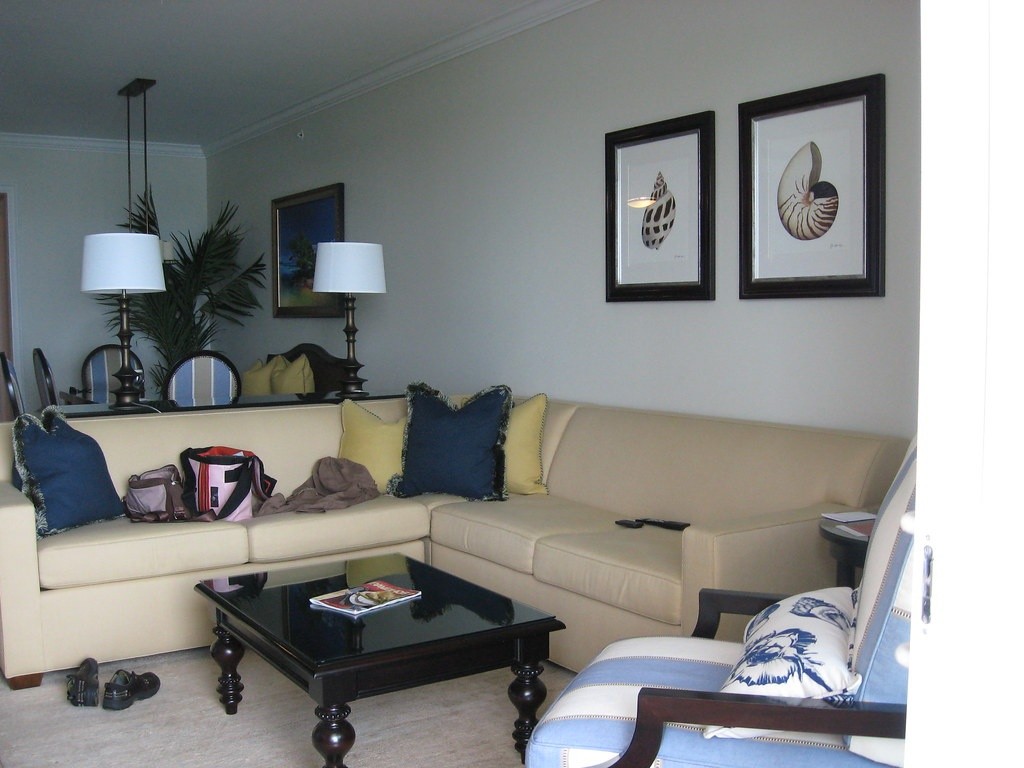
[57,404,156,416]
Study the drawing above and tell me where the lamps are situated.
[313,242,387,398]
[116,79,177,265]
[81,233,166,410]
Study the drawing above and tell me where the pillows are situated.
[244,355,313,394]
[701,587,862,739]
[14,407,126,538]
[337,384,550,502]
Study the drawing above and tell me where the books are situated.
[309,580,422,614]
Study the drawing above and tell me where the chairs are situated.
[525,431,917,767]
[83,345,145,402]
[0,348,59,420]
[164,350,241,407]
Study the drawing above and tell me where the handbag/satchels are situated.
[182,444,277,521]
[123,464,192,523]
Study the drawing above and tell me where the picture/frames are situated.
[604,111,714,301]
[738,72,886,298]
[272,183,344,318]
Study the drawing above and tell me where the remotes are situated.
[643,518,691,531]
[615,519,644,528]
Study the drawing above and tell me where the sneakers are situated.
[104,669,161,710]
[67,658,98,706]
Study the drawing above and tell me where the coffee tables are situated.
[819,513,877,591]
[194,552,567,768]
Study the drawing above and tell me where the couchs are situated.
[425,396,912,676]
[1,393,430,690]
